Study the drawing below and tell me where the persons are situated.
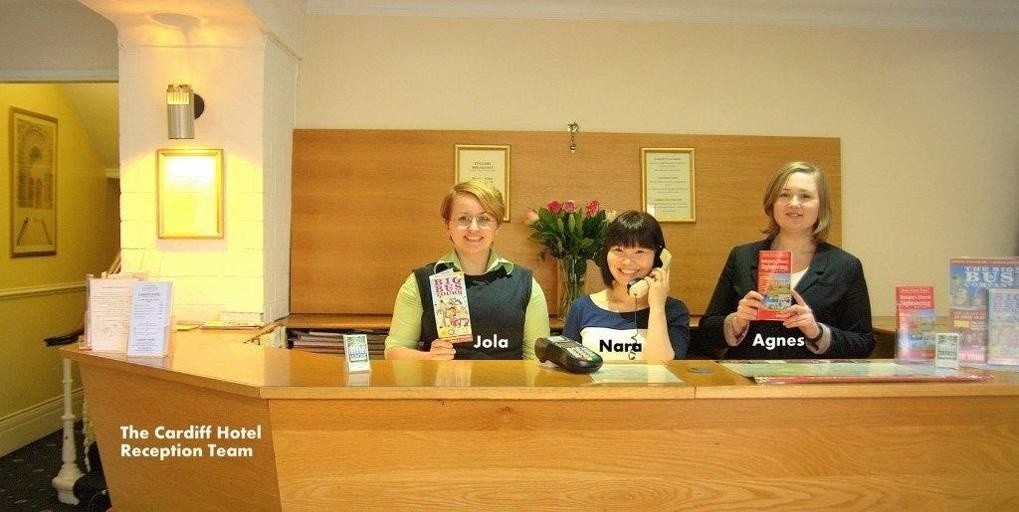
[699,162,874,360]
[563,212,690,362]
[385,181,551,361]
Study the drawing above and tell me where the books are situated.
[289,330,388,355]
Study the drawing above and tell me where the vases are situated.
[556,257,587,321]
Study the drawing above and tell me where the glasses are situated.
[444,213,494,228]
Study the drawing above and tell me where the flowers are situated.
[524,200,610,267]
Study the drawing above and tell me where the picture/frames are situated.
[8,106,58,258]
[637,146,697,224]
[156,149,225,241]
[454,143,512,223]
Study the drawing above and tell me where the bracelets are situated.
[808,322,823,343]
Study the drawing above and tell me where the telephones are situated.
[629,248,673,299]
